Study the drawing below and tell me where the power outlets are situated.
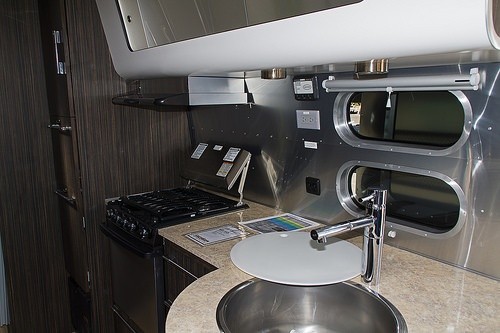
[296,110,321,129]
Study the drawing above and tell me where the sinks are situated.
[215,275,410,333]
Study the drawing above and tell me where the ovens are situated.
[96,220,166,333]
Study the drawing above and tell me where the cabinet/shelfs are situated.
[37,0,218,332]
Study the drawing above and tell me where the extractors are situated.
[112,75,249,112]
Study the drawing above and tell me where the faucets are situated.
[310,206,386,285]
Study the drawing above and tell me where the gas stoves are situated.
[108,185,249,249]
